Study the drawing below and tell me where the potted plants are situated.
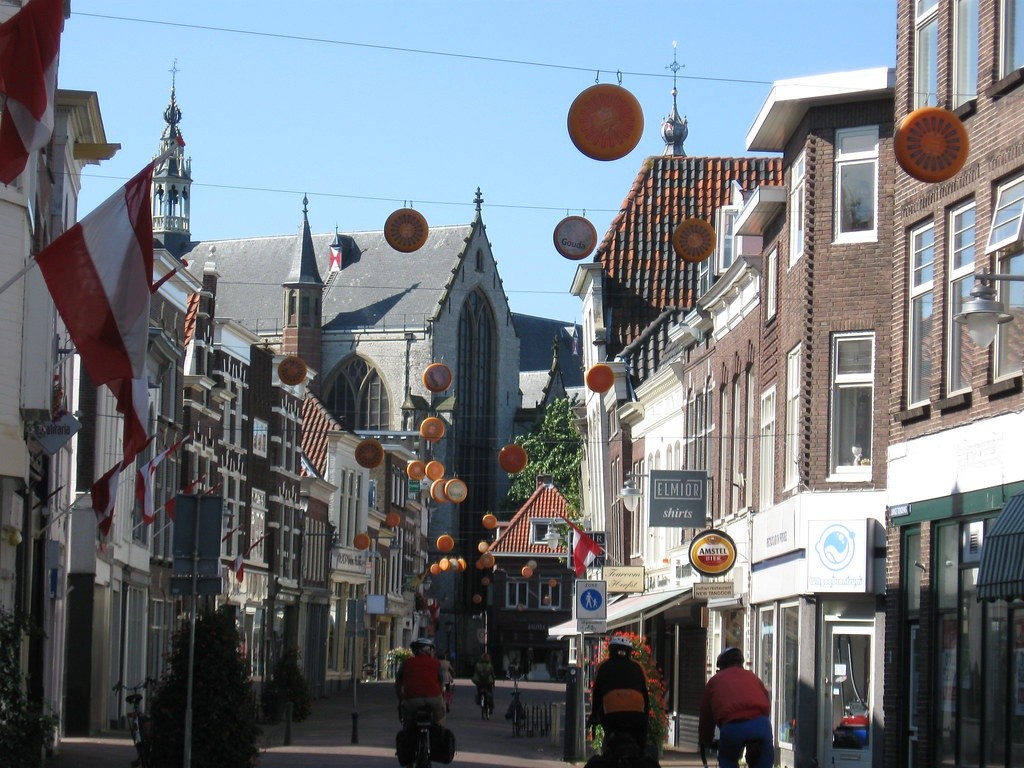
[860,458,871,466]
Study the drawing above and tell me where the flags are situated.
[560,512,602,578]
[0,0,72,189]
[184,289,199,355]
[228,535,264,583]
[411,569,427,590]
[428,597,440,623]
[90,435,218,553]
[35,160,154,471]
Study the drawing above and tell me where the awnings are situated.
[548,586,693,636]
[975,488,1024,603]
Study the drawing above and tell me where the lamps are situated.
[616,471,713,512]
[306,532,344,557]
[370,536,402,557]
[951,267,1024,349]
[541,518,583,550]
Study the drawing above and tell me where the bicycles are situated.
[441,675,455,712]
[504,673,526,738]
[398,691,452,768]
[586,712,655,768]
[112,676,154,768]
[697,738,749,768]
[472,678,496,721]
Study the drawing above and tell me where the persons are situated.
[584,728,661,768]
[395,638,447,728]
[474,653,496,715]
[591,637,651,753]
[435,650,455,713]
[698,647,775,768]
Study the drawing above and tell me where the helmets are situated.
[716,647,745,666]
[608,637,634,651]
[410,638,435,651]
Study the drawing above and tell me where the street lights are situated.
[472,610,489,655]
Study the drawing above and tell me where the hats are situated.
[481,653,490,661]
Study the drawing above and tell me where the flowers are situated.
[585,631,670,756]
[383,646,414,668]
[24,374,68,434]
